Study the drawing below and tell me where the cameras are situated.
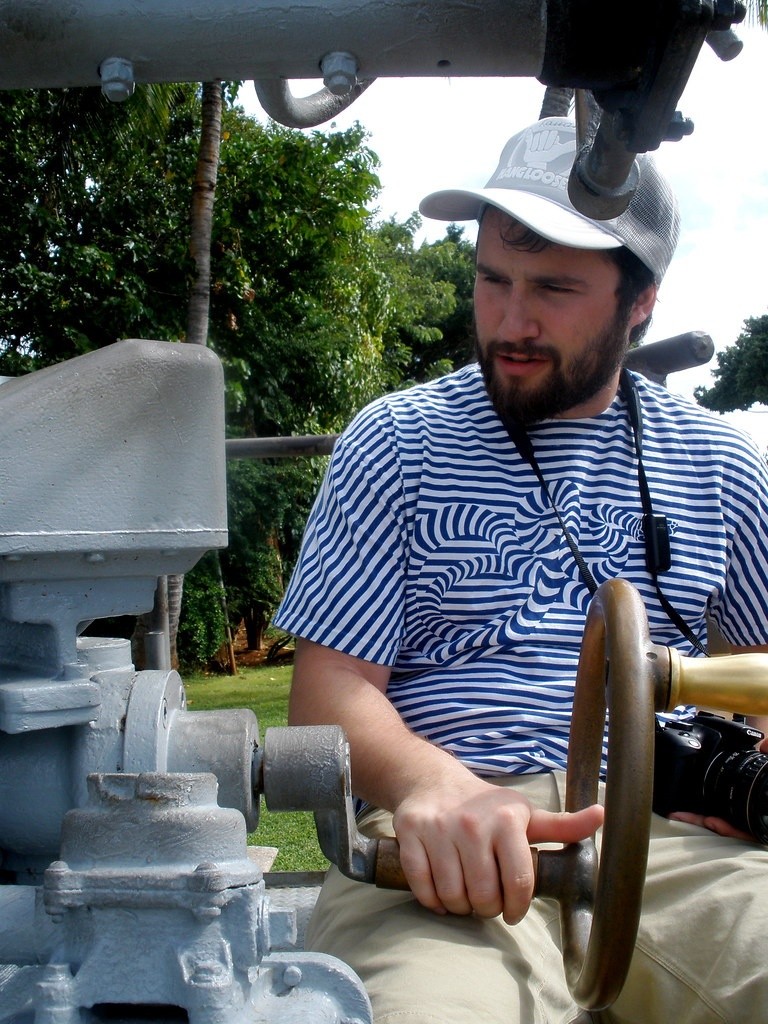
[651,711,768,847]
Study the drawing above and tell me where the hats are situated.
[419,116,680,286]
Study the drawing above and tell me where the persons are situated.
[270,115,768,1024]
